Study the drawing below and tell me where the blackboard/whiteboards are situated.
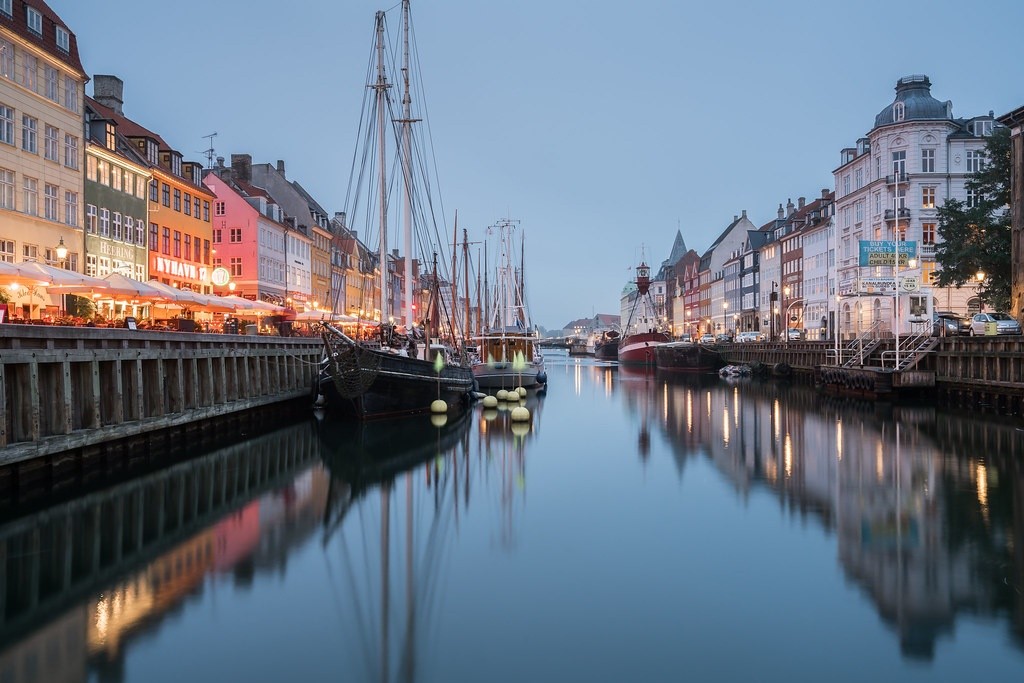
[123,317,137,330]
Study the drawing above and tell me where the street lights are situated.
[56,235,69,317]
[783,285,790,327]
[722,300,729,334]
[975,266,987,314]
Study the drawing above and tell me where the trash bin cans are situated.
[984,321,997,336]
[246,324,258,335]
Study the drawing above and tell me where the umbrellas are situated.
[143,279,207,328]
[209,293,261,335]
[0,260,54,322]
[88,271,160,321]
[294,310,344,337]
[46,260,111,318]
[254,299,288,333]
[354,317,386,342]
[337,314,362,337]
[181,287,237,332]
[16,259,83,322]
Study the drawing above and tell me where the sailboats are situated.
[618,243,674,363]
[316,0,546,429]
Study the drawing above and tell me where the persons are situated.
[727,329,733,343]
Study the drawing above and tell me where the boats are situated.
[654,340,726,371]
[568,329,619,361]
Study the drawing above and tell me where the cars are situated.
[732,331,765,341]
[933,310,1022,337]
[779,329,801,341]
[680,333,693,342]
[698,333,731,343]
